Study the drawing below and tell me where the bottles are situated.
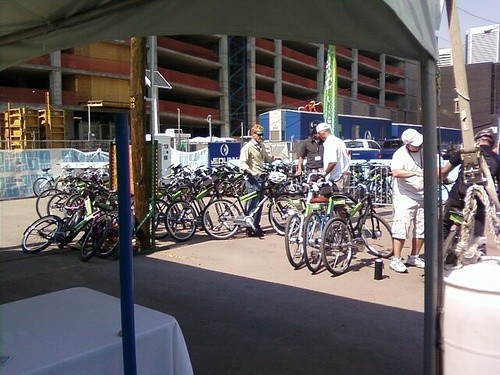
[375,258,383,280]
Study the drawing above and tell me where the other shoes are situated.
[248,231,267,237]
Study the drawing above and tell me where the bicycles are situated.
[21,158,395,276]
[442,180,467,260]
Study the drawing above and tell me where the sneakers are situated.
[390,258,408,272]
[407,255,425,268]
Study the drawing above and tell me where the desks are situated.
[0,286,194,375]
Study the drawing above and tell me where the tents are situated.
[0,0,439,375]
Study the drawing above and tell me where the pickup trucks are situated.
[349,137,403,160]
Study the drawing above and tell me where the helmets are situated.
[310,120,321,129]
[475,129,493,141]
[268,172,287,184]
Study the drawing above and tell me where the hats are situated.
[316,123,331,137]
[401,128,423,147]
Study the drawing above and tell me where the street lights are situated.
[206,114,213,142]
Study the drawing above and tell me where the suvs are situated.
[343,138,382,151]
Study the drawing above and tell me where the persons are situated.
[416,130,500,269]
[238,124,275,237]
[316,123,351,218]
[293,120,328,198]
[390,128,453,273]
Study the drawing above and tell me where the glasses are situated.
[254,132,264,137]
